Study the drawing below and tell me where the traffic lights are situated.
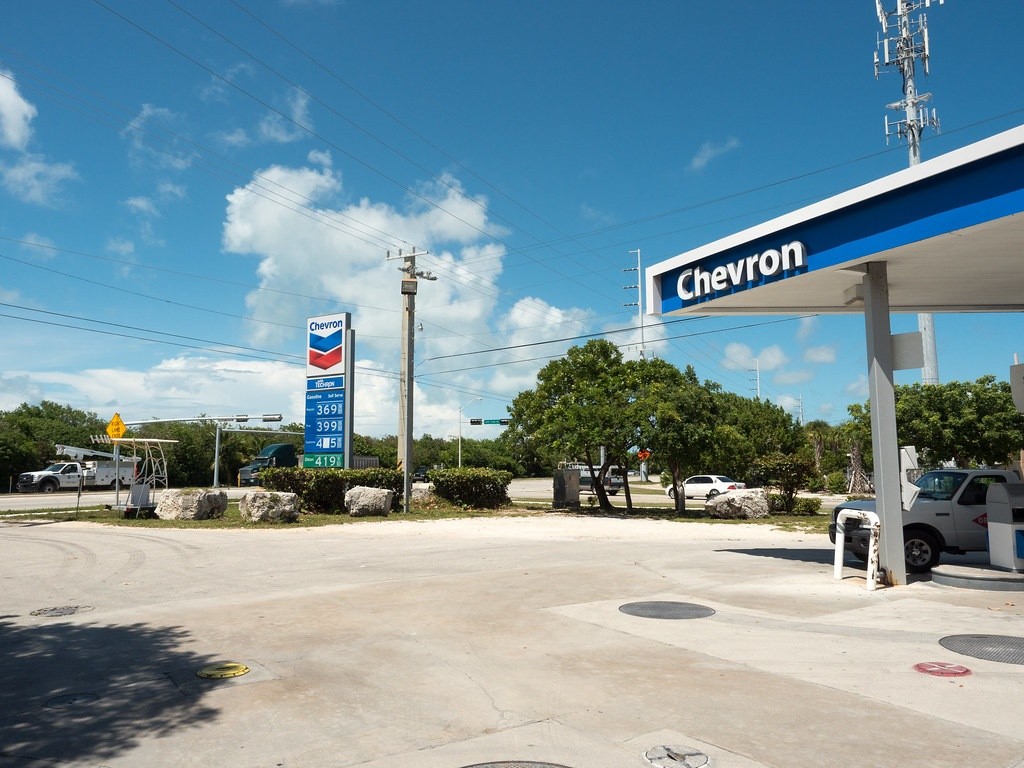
[500,419,510,425]
[470,419,482,425]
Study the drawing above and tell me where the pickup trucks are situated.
[827,465,1024,574]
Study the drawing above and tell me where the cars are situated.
[666,474,747,500]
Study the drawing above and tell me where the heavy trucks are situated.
[238,443,379,489]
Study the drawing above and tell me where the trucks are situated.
[17,444,142,496]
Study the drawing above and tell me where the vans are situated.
[558,462,626,494]
